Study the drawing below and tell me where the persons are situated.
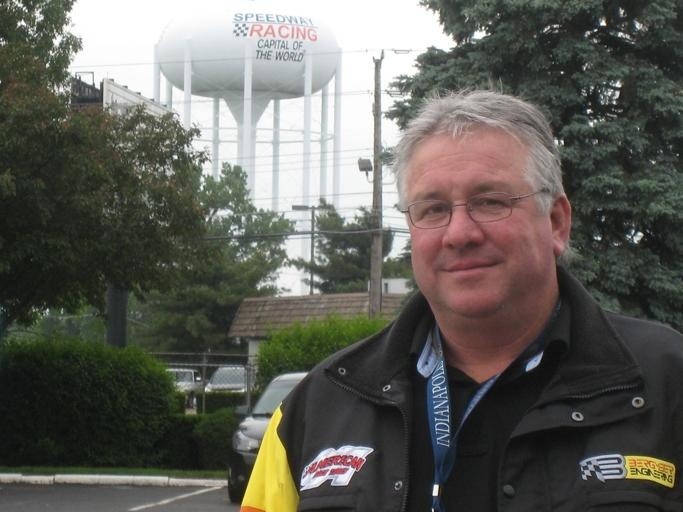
[233,81,683,512]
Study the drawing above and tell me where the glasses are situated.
[396,188,549,229]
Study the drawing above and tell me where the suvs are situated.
[203,365,255,402]
[162,365,204,409]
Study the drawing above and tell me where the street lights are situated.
[292,203,335,295]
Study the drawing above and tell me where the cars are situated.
[224,370,308,504]
